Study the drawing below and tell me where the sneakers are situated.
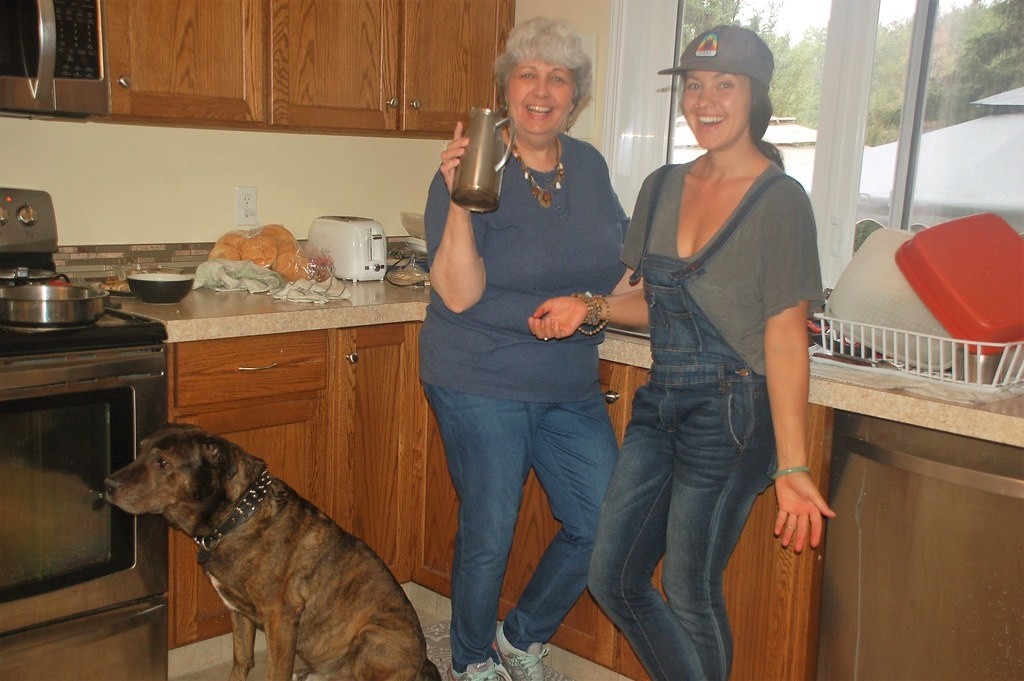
[445,657,507,681]
[493,621,549,681]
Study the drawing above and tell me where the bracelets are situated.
[571,287,612,336]
[768,466,811,480]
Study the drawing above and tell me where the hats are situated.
[656,26,774,91]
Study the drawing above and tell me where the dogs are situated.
[105,423,441,681]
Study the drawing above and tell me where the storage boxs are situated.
[812,312,1024,395]
[826,227,958,370]
[895,212,1024,355]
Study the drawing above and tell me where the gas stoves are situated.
[0,189,168,358]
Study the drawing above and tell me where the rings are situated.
[543,337,548,341]
[785,524,795,530]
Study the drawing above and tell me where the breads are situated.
[207,225,312,280]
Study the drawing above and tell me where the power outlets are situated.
[235,186,258,226]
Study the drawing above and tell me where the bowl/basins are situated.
[824,212,1024,385]
[400,212,425,237]
[126,273,195,305]
[405,237,428,252]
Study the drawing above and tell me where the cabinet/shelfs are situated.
[165,329,349,652]
[92,0,267,133]
[340,321,417,586]
[266,0,516,141]
[411,321,836,681]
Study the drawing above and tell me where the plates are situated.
[98,284,136,297]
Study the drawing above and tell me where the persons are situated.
[527,26,836,680]
[419,15,632,681]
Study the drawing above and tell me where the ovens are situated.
[0,0,109,115]
[0,344,168,681]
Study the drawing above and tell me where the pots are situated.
[0,284,122,333]
[0,267,71,284]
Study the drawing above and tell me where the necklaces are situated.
[499,123,569,205]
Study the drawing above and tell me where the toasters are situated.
[306,216,387,282]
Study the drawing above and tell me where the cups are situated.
[451,106,517,212]
